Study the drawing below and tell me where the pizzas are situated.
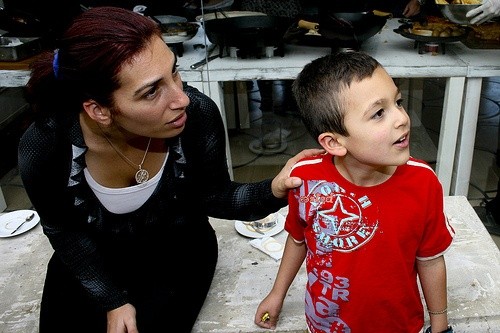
[413,16,457,30]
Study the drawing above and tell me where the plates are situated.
[0,210,41,238]
[235,213,285,238]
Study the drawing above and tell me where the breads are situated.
[467,22,500,40]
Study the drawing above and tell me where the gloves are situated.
[466,0,500,27]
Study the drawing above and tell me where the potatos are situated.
[433,27,461,37]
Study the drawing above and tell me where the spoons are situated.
[11,213,34,234]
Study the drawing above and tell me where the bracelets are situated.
[425,325,453,333]
[428,307,448,315]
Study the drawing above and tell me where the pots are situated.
[146,0,483,60]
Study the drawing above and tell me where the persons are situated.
[254,52,455,333]
[466,0,500,26]
[15,8,325,333]
[473,124,500,238]
[402,0,426,17]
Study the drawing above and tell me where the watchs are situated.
[418,0,425,5]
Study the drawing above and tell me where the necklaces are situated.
[93,119,151,184]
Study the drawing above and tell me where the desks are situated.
[0,20,500,333]
